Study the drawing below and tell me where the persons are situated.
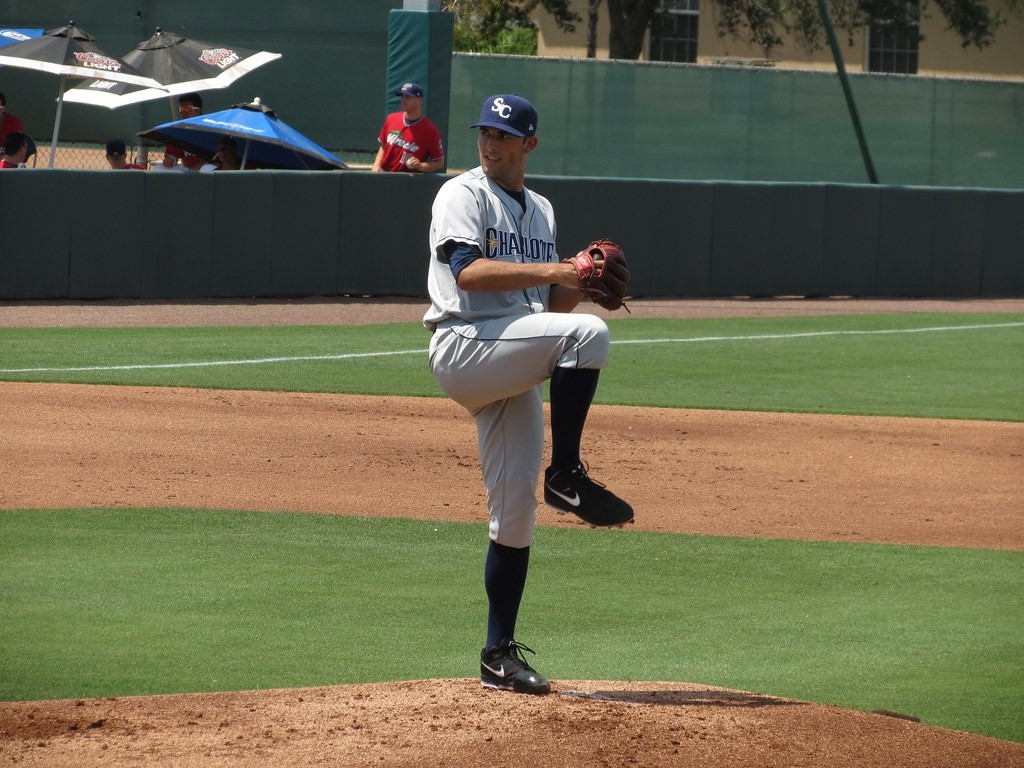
[370,82,445,172]
[0,90,255,172]
[421,92,637,696]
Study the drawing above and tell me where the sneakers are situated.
[544,458,634,527]
[480,639,550,695]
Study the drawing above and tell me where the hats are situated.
[396,83,423,98]
[469,92,537,138]
[106,139,126,156]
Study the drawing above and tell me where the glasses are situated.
[178,106,199,113]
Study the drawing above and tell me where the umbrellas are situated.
[135,95,352,170]
[55,26,283,109]
[0,20,172,95]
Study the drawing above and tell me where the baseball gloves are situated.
[564,237,631,310]
